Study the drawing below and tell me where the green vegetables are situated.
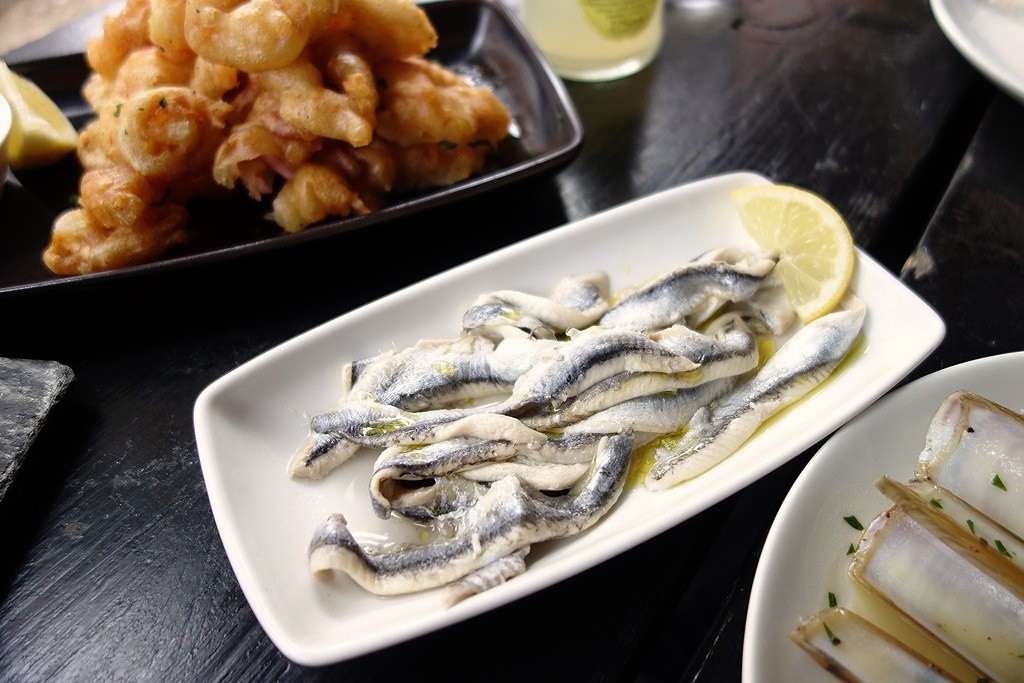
[821,474,1010,646]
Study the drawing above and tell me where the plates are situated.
[741,351,1024,683]
[929,1,1024,102]
[192,171,946,667]
[0,2,583,307]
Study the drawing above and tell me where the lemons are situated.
[736,186,856,325]
[0,59,81,169]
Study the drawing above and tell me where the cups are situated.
[518,0,665,81]
